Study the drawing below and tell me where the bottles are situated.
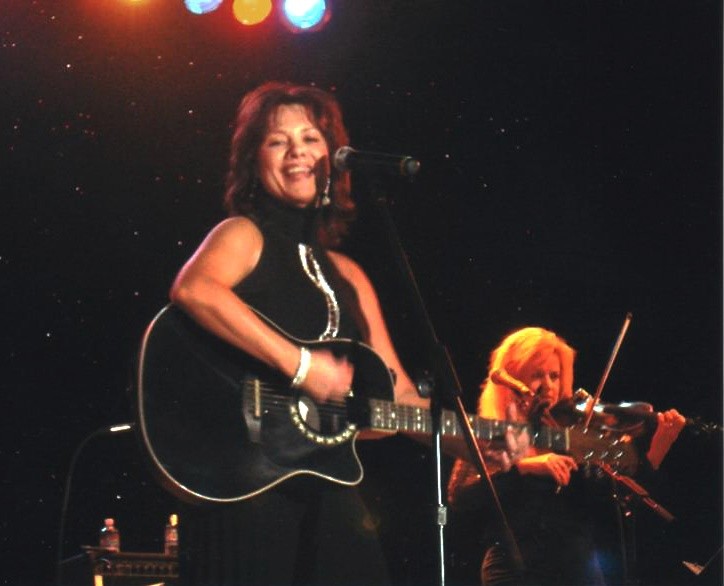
[99,518,120,553]
[164,513,179,554]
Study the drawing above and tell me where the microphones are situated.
[332,144,422,178]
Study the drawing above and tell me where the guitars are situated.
[140,292,637,507]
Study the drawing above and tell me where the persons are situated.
[444,325,687,586]
[140,80,532,586]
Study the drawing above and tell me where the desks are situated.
[57,547,181,586]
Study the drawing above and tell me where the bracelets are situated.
[291,348,311,389]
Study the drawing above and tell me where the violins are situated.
[548,398,719,440]
